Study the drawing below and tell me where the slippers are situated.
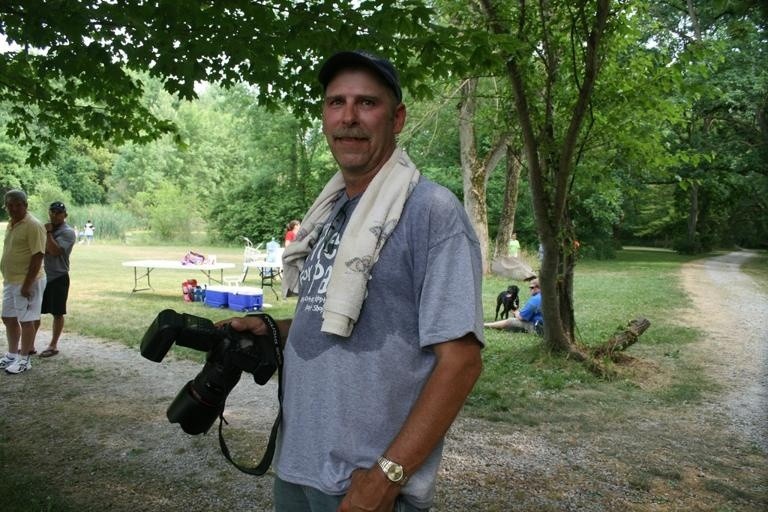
[39,350,59,357]
[17,349,37,355]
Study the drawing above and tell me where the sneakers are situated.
[5,355,32,373]
[0,352,18,369]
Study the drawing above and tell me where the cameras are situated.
[136,309,279,436]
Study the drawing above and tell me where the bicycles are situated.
[237,234,284,283]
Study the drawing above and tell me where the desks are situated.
[243,262,282,301]
[121,259,235,298]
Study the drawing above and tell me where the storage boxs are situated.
[203,284,262,313]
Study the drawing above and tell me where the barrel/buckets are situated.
[266,237,281,266]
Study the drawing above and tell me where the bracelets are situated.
[47,231,52,233]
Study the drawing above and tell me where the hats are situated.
[529,278,540,288]
[50,202,64,212]
[319,51,402,101]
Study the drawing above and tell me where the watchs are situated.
[377,455,409,487]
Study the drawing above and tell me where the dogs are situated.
[495,285,520,321]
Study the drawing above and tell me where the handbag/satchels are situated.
[181,251,204,265]
[182,280,197,301]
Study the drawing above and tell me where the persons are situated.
[0,189,49,374]
[506,232,522,258]
[484,278,545,338]
[213,51,488,511]
[83,220,96,246]
[18,202,77,357]
[284,220,301,248]
